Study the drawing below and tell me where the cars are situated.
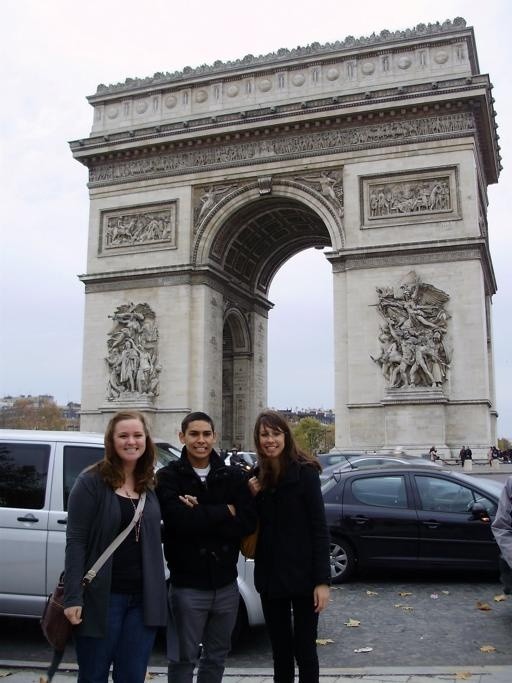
[305,450,365,470]
[314,453,464,498]
[300,467,512,588]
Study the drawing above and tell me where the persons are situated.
[242,412,331,683]
[369,180,450,217]
[154,413,256,683]
[374,284,450,389]
[107,211,171,245]
[488,446,503,467]
[430,446,439,462]
[294,170,344,219]
[490,476,512,595]
[103,312,162,401]
[194,183,234,227]
[62,411,167,683]
[460,445,472,467]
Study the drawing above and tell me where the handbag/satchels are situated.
[501,558,512,594]
[39,571,86,652]
[230,453,260,559]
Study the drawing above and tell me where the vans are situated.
[0,424,296,656]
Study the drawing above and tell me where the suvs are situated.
[228,451,258,469]
